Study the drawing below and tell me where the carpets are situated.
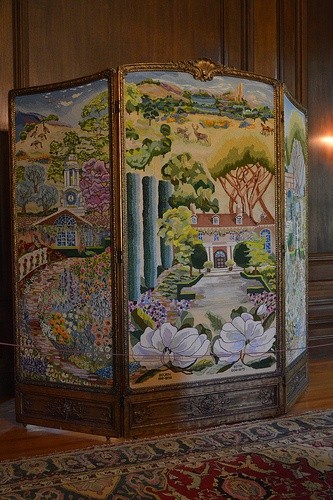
[0,409,333,500]
